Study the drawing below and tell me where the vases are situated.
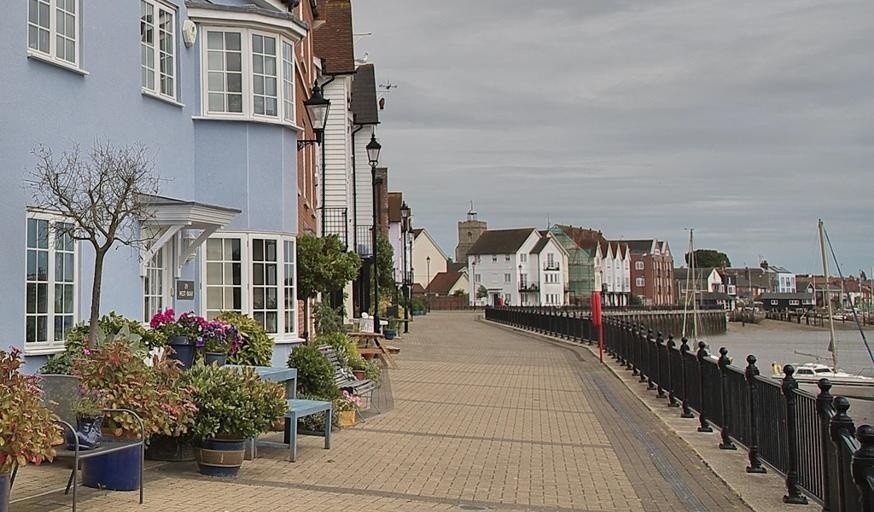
[338,407,356,427]
[166,336,227,369]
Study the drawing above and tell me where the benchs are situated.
[356,344,394,370]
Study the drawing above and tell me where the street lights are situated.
[400,200,409,333]
[427,256,430,313]
[365,126,382,333]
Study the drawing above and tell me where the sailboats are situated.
[770,218,873,402]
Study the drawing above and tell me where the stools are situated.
[251,398,331,462]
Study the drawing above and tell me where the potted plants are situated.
[181,355,290,478]
[1,346,66,510]
[37,310,198,490]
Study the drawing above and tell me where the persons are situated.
[772,361,781,375]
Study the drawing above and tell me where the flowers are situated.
[336,388,362,411]
[150,302,244,354]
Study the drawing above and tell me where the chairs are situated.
[13,370,145,511]
[318,345,382,422]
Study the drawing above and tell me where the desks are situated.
[218,365,297,463]
[346,332,398,370]
